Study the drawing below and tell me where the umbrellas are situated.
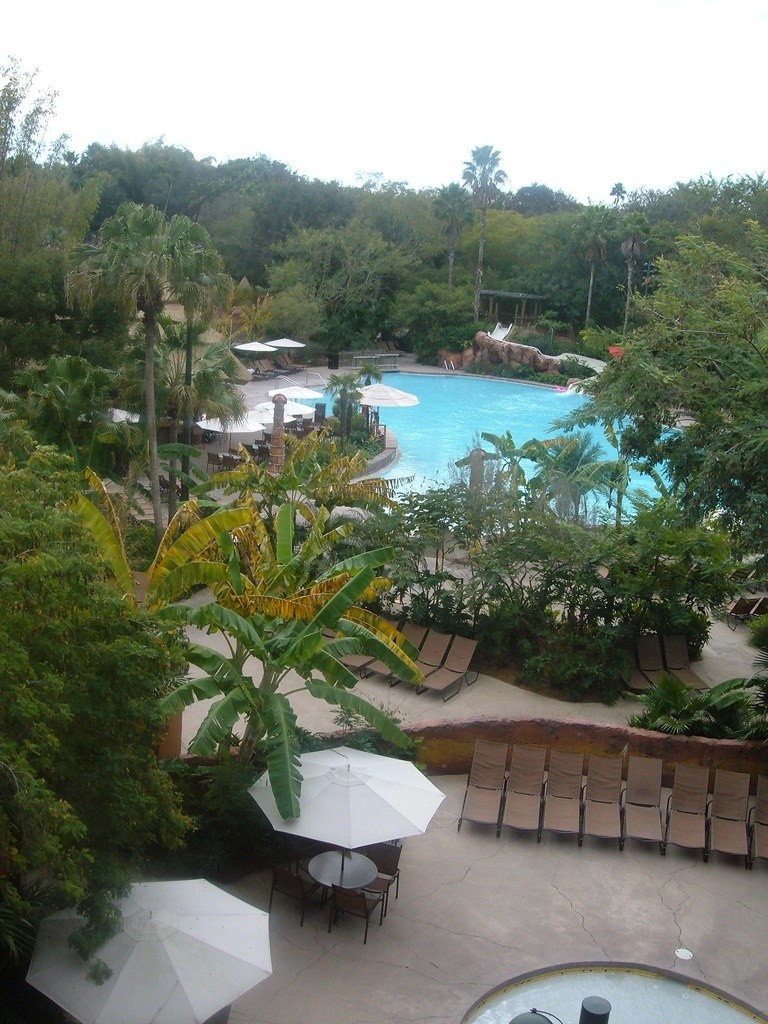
[337,382,421,429]
[234,341,278,362]
[25,876,274,1024]
[268,386,324,403]
[246,744,447,870]
[254,399,317,415]
[78,407,140,424]
[196,415,267,450]
[266,338,305,348]
[242,408,297,440]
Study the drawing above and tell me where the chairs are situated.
[497,744,547,842]
[246,354,308,382]
[747,773,768,870]
[264,848,325,927]
[706,769,750,870]
[616,595,768,699]
[361,842,403,917]
[364,621,429,686]
[538,747,585,847]
[328,883,385,944]
[388,630,453,696]
[663,763,710,863]
[457,739,509,837]
[222,414,330,471]
[144,469,183,503]
[416,635,481,703]
[620,756,664,856]
[580,752,623,851]
[321,628,338,642]
[339,619,401,679]
[206,452,225,473]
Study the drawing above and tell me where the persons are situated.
[500,303,513,321]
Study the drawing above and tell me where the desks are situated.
[307,850,379,914]
[251,443,272,455]
[217,453,242,465]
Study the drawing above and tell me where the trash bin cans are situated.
[328,353,339,368]
